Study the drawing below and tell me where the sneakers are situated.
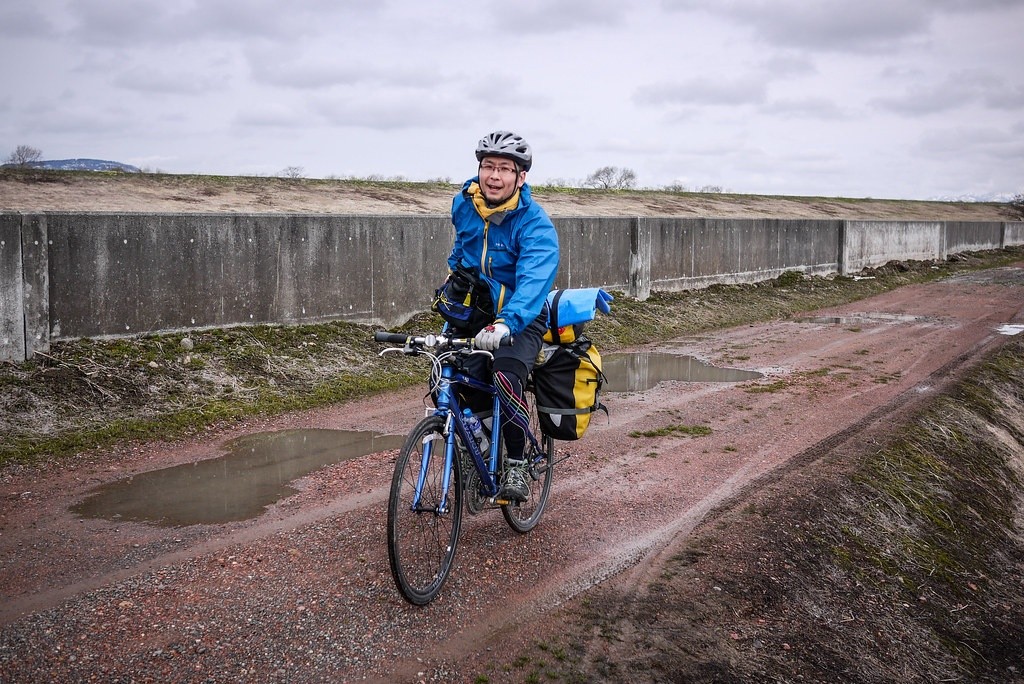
[460,451,477,489]
[501,457,529,502]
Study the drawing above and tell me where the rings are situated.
[483,323,495,334]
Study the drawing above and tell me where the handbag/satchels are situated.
[534,336,609,441]
[433,262,494,332]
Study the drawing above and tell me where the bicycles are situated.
[370,323,571,606]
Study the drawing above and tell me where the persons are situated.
[427,133,560,502]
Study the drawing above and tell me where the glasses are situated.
[479,165,523,174]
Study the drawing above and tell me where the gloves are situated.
[475,323,510,349]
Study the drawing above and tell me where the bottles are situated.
[462,408,489,453]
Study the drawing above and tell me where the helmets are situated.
[475,130,533,175]
